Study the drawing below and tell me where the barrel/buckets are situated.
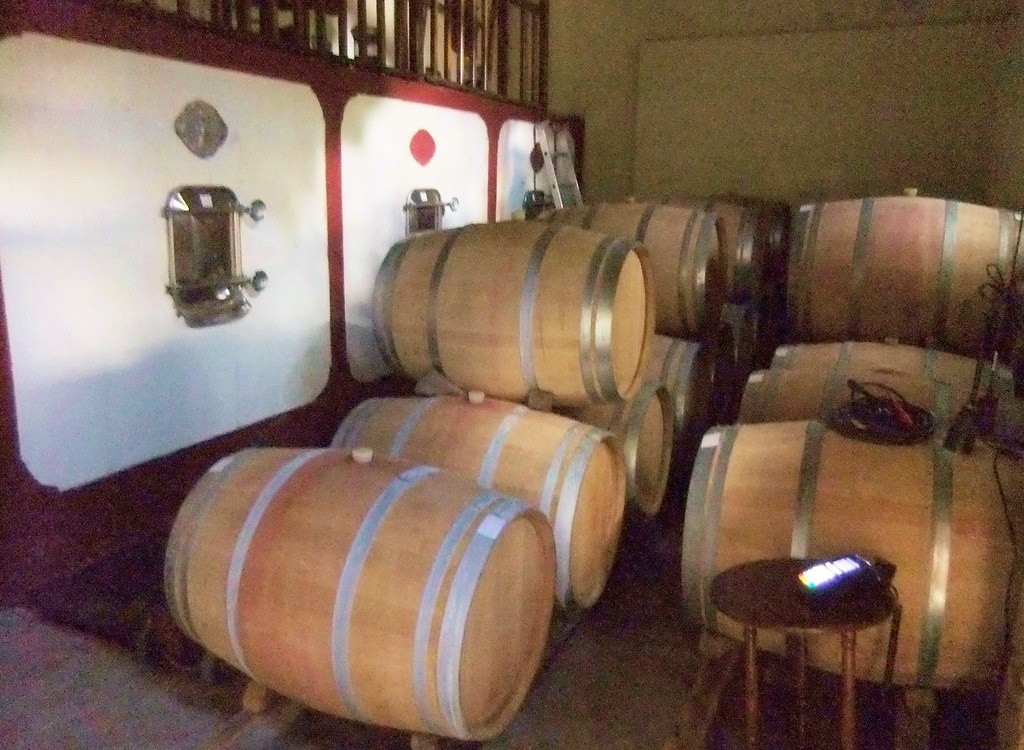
[161,191,1023,736]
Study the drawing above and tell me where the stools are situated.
[706,559,904,750]
